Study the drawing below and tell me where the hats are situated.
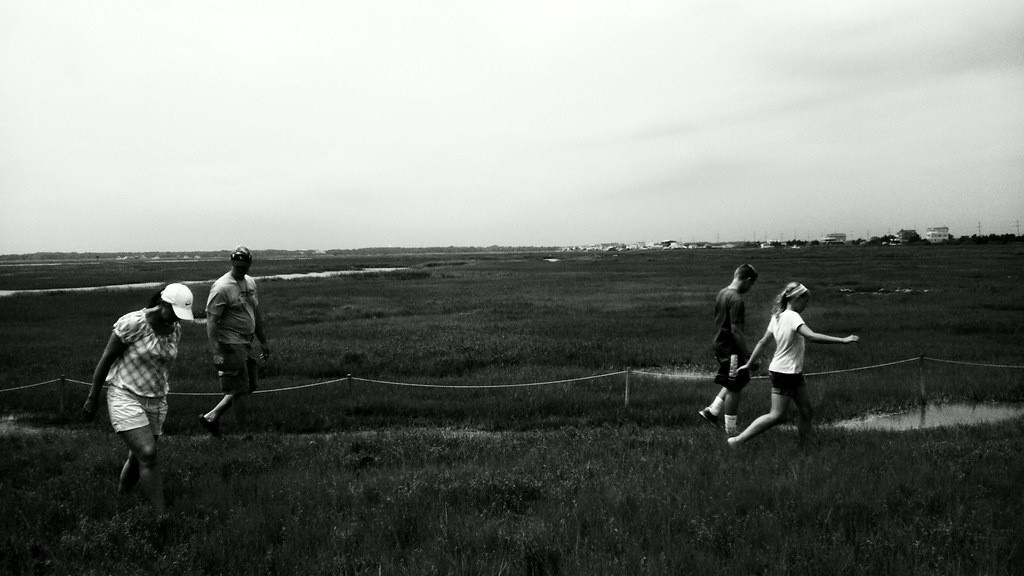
[161,282,194,322]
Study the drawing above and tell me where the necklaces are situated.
[232,274,248,302]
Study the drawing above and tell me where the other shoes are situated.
[726,435,745,452]
[699,403,739,434]
[197,414,257,442]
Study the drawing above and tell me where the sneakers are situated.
[116,490,183,522]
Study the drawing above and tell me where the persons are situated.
[83,283,195,525]
[697,264,759,437]
[197,246,269,439]
[727,282,860,451]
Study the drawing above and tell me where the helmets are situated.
[231,250,253,269]
